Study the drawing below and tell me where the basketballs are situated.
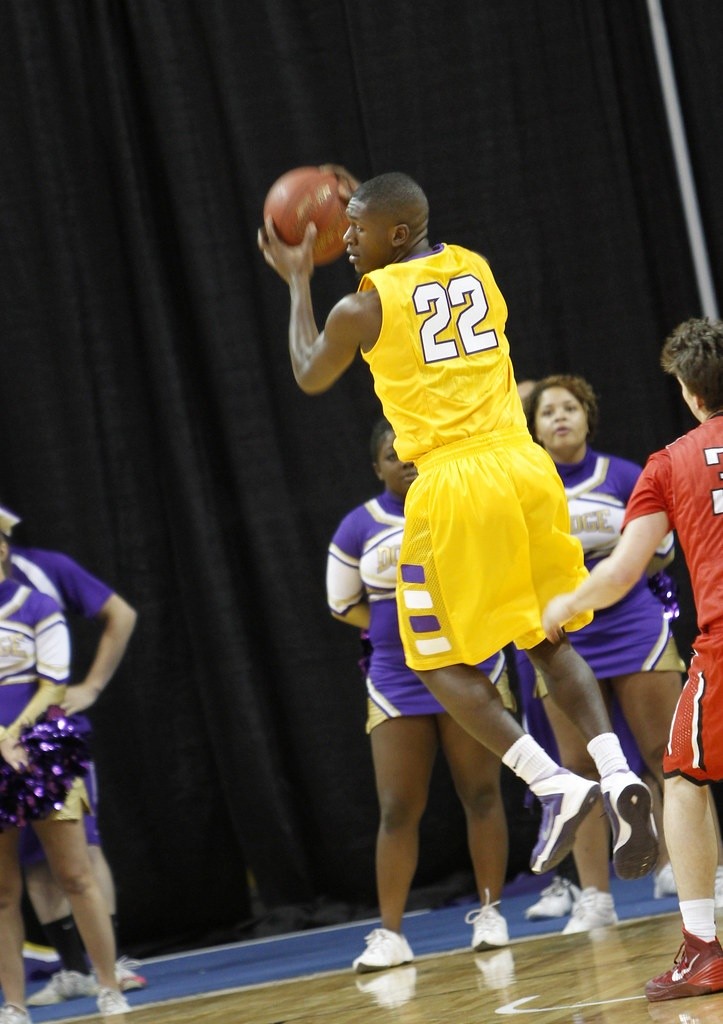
[264,165,352,267]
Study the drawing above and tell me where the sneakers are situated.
[561,884,619,936]
[58,970,100,997]
[113,963,143,991]
[128,968,145,985]
[95,985,129,1012]
[524,874,581,921]
[471,904,509,952]
[0,1002,31,1024]
[601,769,661,881]
[645,924,722,1002]
[714,864,723,909]
[24,968,67,1006]
[351,927,415,974]
[653,859,679,900]
[528,764,601,876]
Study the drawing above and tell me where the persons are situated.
[259,165,657,881]
[544,317,723,1001]
[327,419,516,972]
[517,374,722,935]
[0,510,145,1024]
[354,948,523,1024]
